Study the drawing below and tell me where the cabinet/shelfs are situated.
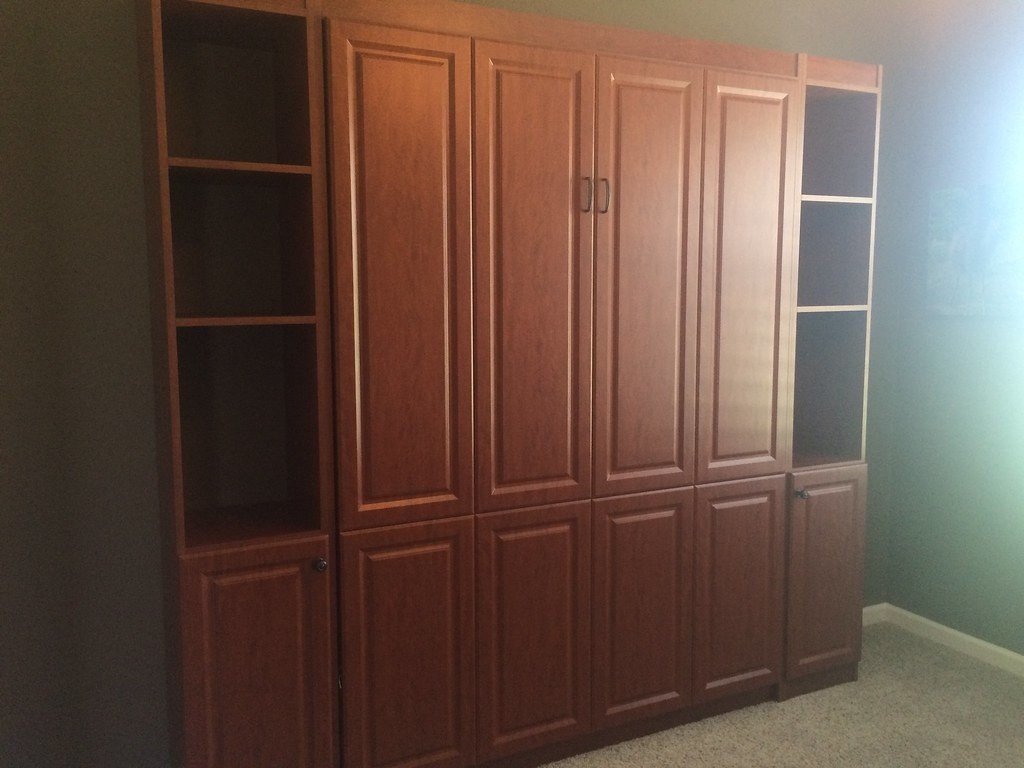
[788,48,887,698]
[148,0,338,768]
[340,474,790,768]
[334,0,790,529]
